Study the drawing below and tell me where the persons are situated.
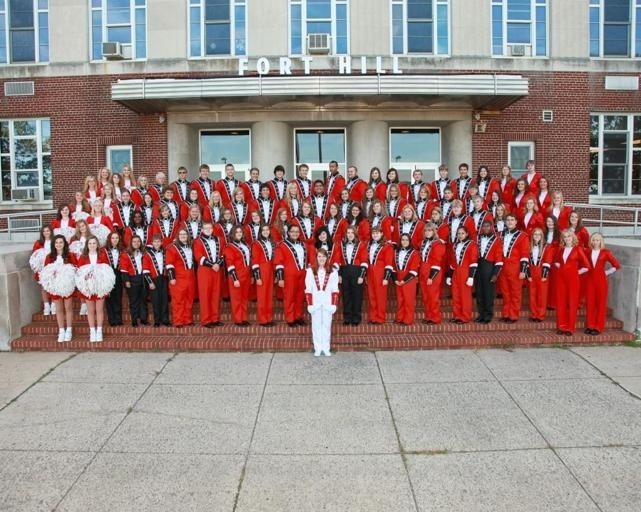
[584,231,622,337]
[43,234,76,344]
[553,227,591,337]
[304,248,340,358]
[30,159,592,329]
[73,235,109,342]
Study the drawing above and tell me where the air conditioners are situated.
[511,44,526,56]
[101,42,125,58]
[305,31,330,55]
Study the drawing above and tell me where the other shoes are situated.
[109,317,307,331]
[342,319,541,324]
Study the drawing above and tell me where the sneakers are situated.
[90,332,104,342]
[314,348,332,357]
[80,307,89,315]
[58,333,73,343]
[557,328,600,336]
[44,307,57,315]
